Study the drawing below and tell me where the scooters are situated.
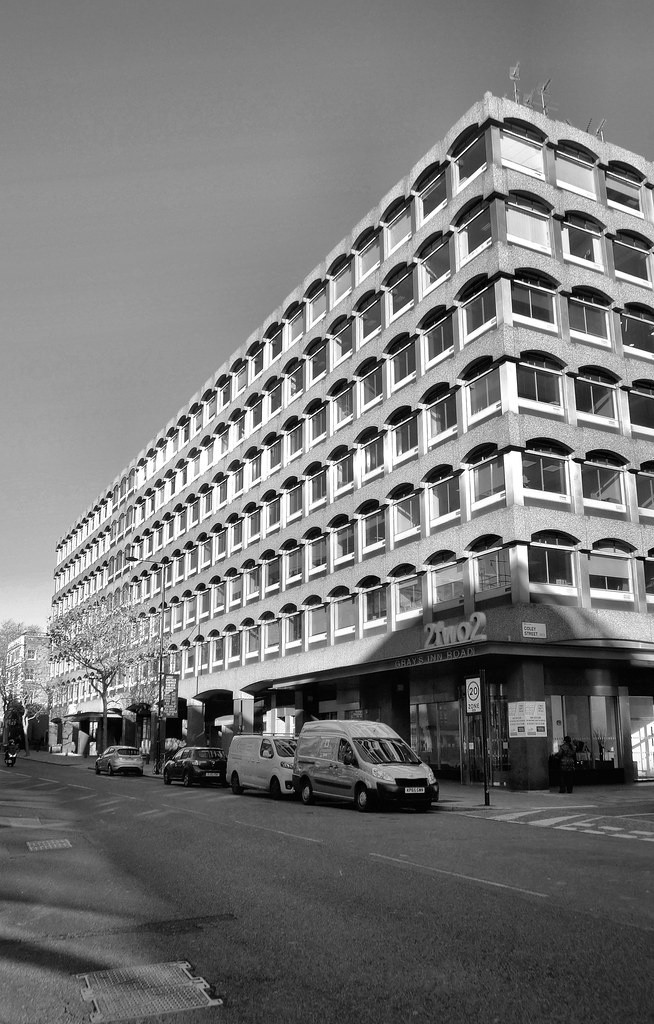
[3,743,19,766]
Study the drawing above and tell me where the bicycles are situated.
[151,752,165,775]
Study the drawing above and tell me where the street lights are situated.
[127,557,171,761]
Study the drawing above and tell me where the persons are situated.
[5,739,16,759]
[556,735,579,794]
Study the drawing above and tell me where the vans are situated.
[225,733,301,801]
[292,720,441,812]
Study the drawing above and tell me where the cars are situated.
[163,746,228,787]
[95,745,144,777]
